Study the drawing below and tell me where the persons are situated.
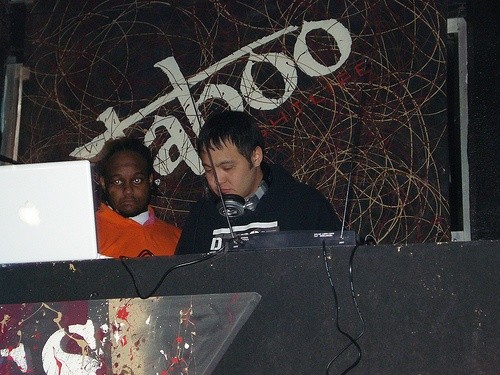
[95,137,183,259]
[173,111,346,256]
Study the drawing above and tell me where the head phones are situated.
[204,181,269,218]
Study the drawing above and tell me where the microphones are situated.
[360,234,377,245]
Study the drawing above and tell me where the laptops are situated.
[0,159,113,265]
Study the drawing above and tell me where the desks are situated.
[0,240,500,374]
